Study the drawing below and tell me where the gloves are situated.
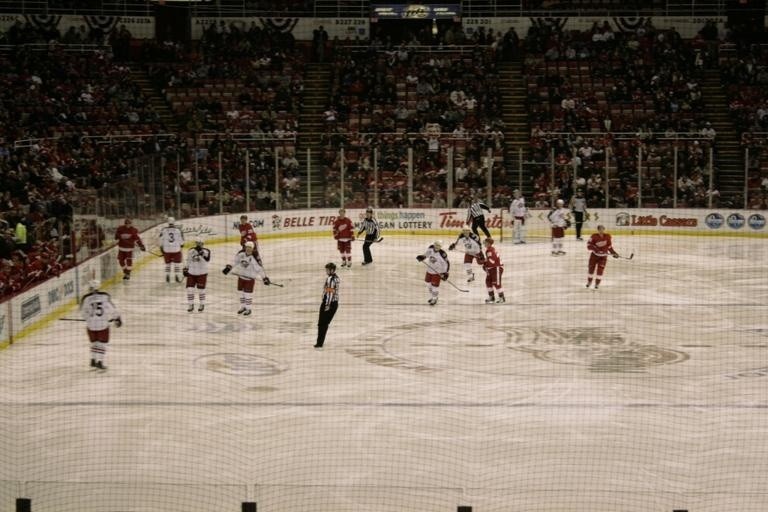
[182,268,190,276]
[195,245,204,256]
[612,252,619,258]
[416,253,427,263]
[261,276,270,286]
[114,316,123,327]
[139,245,147,252]
[442,271,450,281]
[222,264,233,275]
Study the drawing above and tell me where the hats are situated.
[576,187,584,192]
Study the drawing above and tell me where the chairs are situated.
[0,38,304,210]
[741,109,768,209]
[320,37,509,208]
[522,37,721,210]
[716,37,767,108]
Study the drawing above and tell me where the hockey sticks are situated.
[355,238,384,243]
[134,244,164,257]
[609,253,635,261]
[423,260,470,294]
[571,214,589,224]
[229,271,291,288]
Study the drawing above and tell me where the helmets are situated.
[462,223,469,230]
[484,238,495,244]
[597,224,605,230]
[195,237,201,243]
[338,208,346,214]
[87,280,99,289]
[365,207,373,212]
[124,218,132,224]
[167,216,175,225]
[324,262,337,269]
[240,214,248,221]
[556,199,564,204]
[244,241,255,249]
[433,239,443,248]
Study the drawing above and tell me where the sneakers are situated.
[95,360,107,369]
[197,304,205,311]
[512,236,585,257]
[242,309,252,316]
[187,304,195,312]
[585,281,592,288]
[121,273,131,279]
[427,297,438,306]
[165,273,170,283]
[174,274,180,283]
[594,283,599,289]
[236,307,246,315]
[340,260,373,267]
[88,357,97,368]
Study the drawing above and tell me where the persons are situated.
[313,263,340,348]
[78,279,122,376]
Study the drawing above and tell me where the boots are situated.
[496,292,506,304]
[484,290,496,303]
[465,272,476,282]
